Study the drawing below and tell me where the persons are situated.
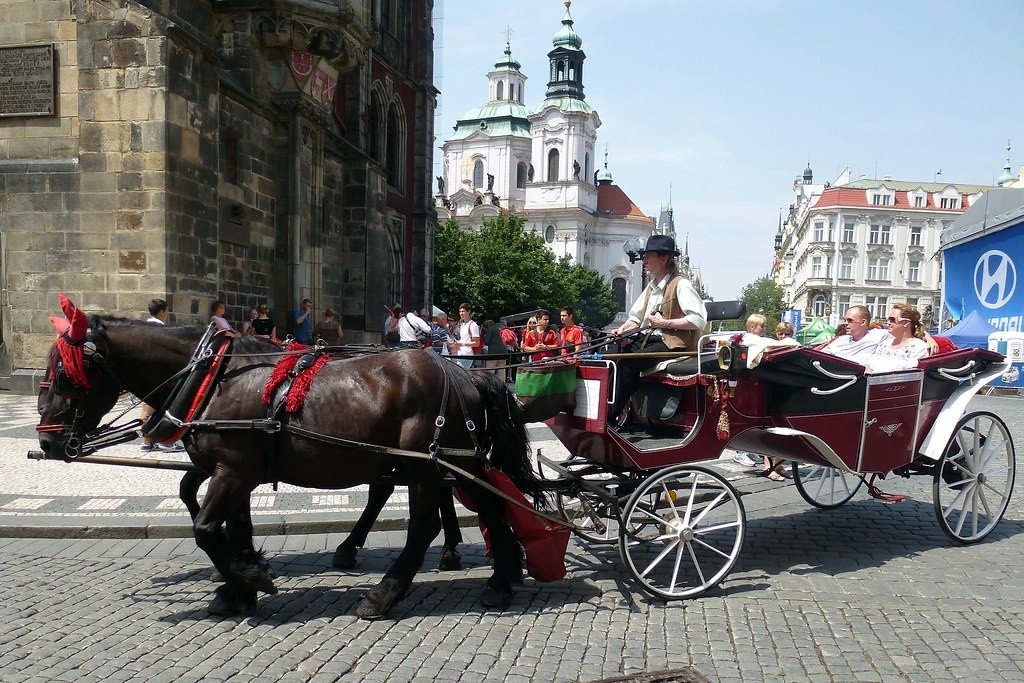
[813,303,940,375]
[608,235,707,427]
[295,298,312,343]
[209,301,242,339]
[315,310,343,345]
[386,303,607,382]
[243,303,276,339]
[140,299,185,454]
[733,313,796,481]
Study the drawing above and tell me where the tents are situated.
[796,317,837,346]
[808,327,836,345]
[937,309,1001,344]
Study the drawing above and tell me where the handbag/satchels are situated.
[499,326,516,345]
[387,318,400,344]
[442,343,451,361]
[415,329,428,343]
[468,321,484,353]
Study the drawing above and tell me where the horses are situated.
[37,292,555,622]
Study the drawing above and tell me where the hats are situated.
[637,235,680,256]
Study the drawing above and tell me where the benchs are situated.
[648,300,747,375]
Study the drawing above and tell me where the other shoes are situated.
[767,471,785,481]
[775,467,794,479]
[505,379,513,383]
[162,444,185,452]
[140,444,161,451]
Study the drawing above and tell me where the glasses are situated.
[777,332,790,336]
[887,317,913,323]
[844,318,863,323]
[448,319,454,322]
[751,323,765,328]
